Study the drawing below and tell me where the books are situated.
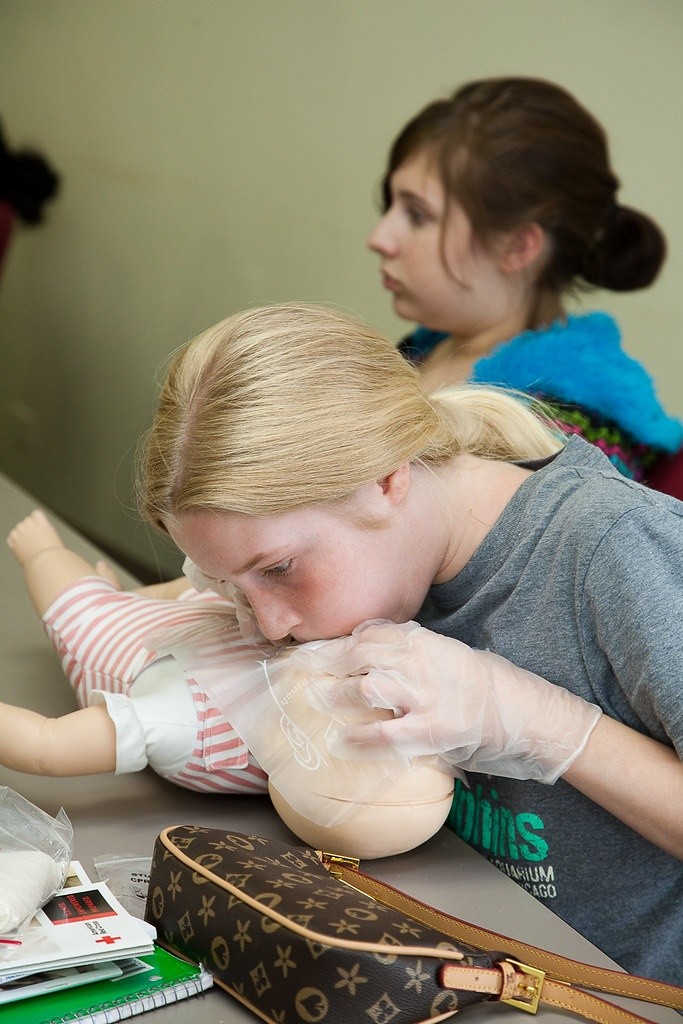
[0,859,214,1023]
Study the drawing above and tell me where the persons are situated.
[0,505,452,859]
[363,74,681,494]
[129,296,683,1005]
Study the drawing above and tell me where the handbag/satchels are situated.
[144,824,683,1024]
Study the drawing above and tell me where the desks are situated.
[0,472,683,1024]
